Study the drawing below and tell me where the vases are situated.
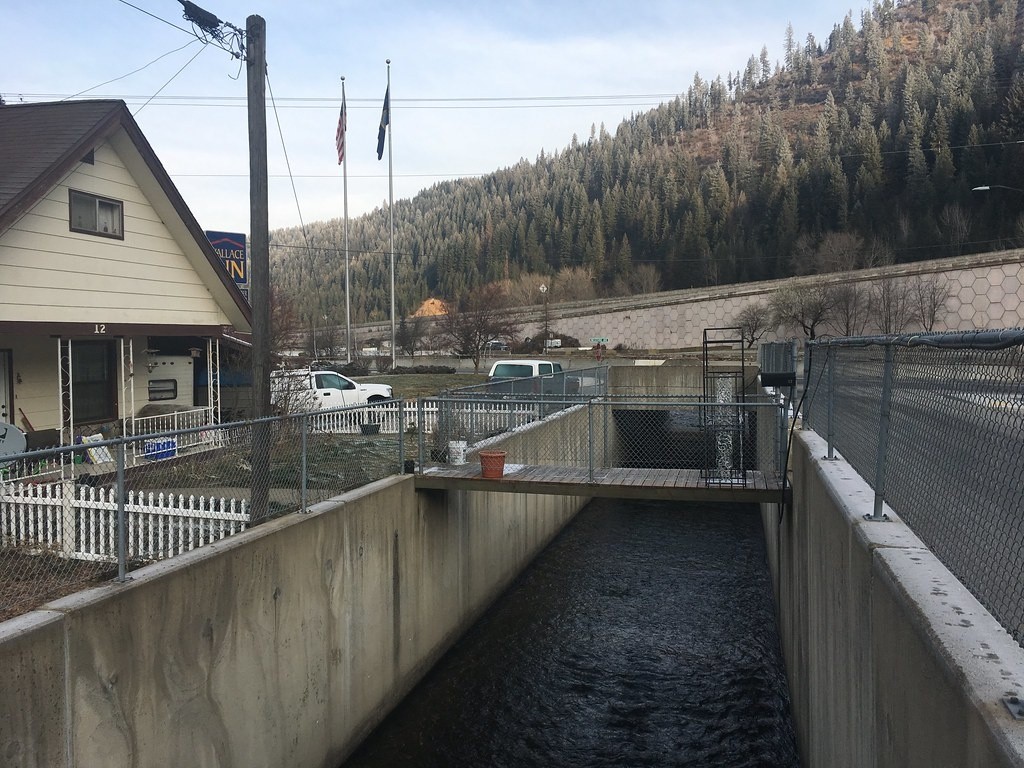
[478,450,507,477]
[360,424,380,435]
[431,449,448,463]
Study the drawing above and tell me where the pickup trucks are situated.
[269,367,396,424]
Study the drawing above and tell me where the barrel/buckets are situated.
[479,450,507,478]
[449,440,468,466]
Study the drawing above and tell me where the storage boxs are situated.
[143,439,176,460]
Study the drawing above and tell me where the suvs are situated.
[485,360,573,413]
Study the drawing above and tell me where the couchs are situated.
[121,403,208,454]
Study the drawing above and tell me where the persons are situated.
[594,343,602,361]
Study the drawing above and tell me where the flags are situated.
[376,85,390,160]
[336,92,346,165]
